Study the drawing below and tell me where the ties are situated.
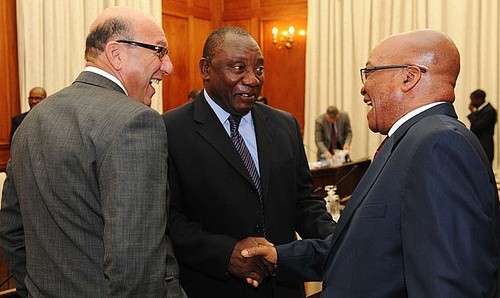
[330,123,337,155]
[226,113,265,236]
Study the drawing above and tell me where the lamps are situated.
[271,24,294,49]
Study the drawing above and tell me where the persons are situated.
[0,5,186,298]
[162,25,341,298]
[256,96,268,105]
[9,86,47,151]
[466,89,498,171]
[315,106,355,164]
[187,89,202,102]
[240,28,500,298]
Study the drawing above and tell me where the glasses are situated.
[360,64,426,85]
[115,39,169,62]
[27,95,45,101]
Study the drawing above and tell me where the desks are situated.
[310,158,371,204]
